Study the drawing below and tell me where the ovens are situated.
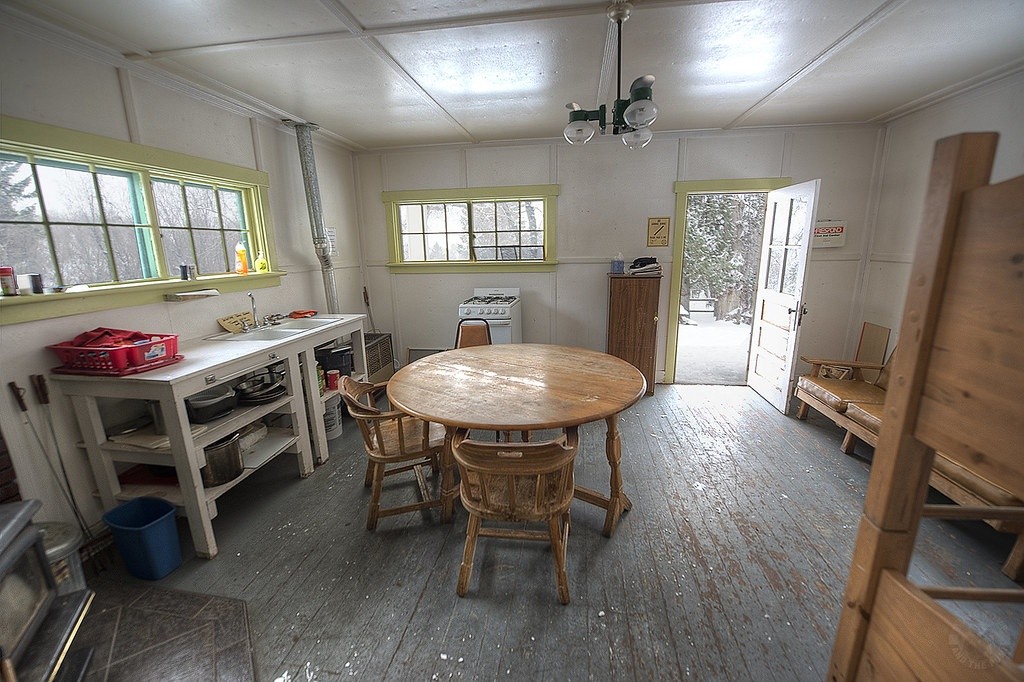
[487,320,523,345]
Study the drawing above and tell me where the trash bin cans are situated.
[339,332,395,384]
[30,520,86,596]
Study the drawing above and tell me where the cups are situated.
[327,370,340,390]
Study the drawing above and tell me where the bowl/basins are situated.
[53,287,67,293]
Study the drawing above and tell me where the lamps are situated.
[563,3,658,148]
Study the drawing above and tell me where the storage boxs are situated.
[46,332,178,371]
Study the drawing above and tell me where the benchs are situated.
[793,344,1024,582]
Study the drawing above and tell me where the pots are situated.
[200,431,244,489]
[183,370,287,425]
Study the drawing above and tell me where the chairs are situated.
[451,425,580,606]
[338,374,462,534]
[454,318,492,350]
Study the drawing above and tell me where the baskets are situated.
[46,328,178,372]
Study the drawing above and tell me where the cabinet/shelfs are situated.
[53,313,366,560]
[606,271,665,396]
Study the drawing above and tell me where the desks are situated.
[387,343,647,537]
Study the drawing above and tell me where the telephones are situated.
[633,256,657,267]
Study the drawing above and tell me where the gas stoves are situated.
[458,287,521,319]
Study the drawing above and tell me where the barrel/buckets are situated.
[305,394,343,441]
[102,496,183,581]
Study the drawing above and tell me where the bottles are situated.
[180,262,187,280]
[188,263,197,280]
[0,266,18,296]
[234,241,248,276]
